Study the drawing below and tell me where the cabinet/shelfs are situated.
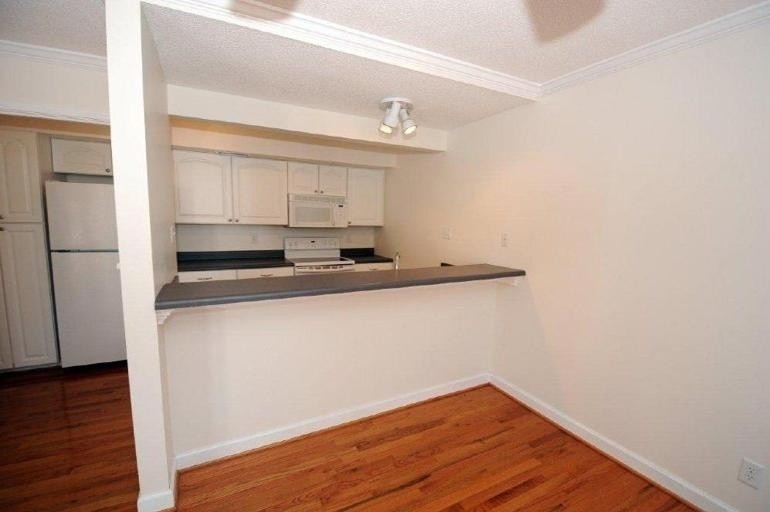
[176,149,290,227]
[291,163,348,199]
[51,135,113,176]
[347,169,390,227]
[1,127,68,374]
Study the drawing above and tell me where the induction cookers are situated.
[287,256,355,266]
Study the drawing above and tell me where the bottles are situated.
[394,251,401,272]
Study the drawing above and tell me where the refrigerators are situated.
[44,181,126,369]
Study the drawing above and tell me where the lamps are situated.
[378,97,418,135]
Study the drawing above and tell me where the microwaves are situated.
[290,193,349,229]
